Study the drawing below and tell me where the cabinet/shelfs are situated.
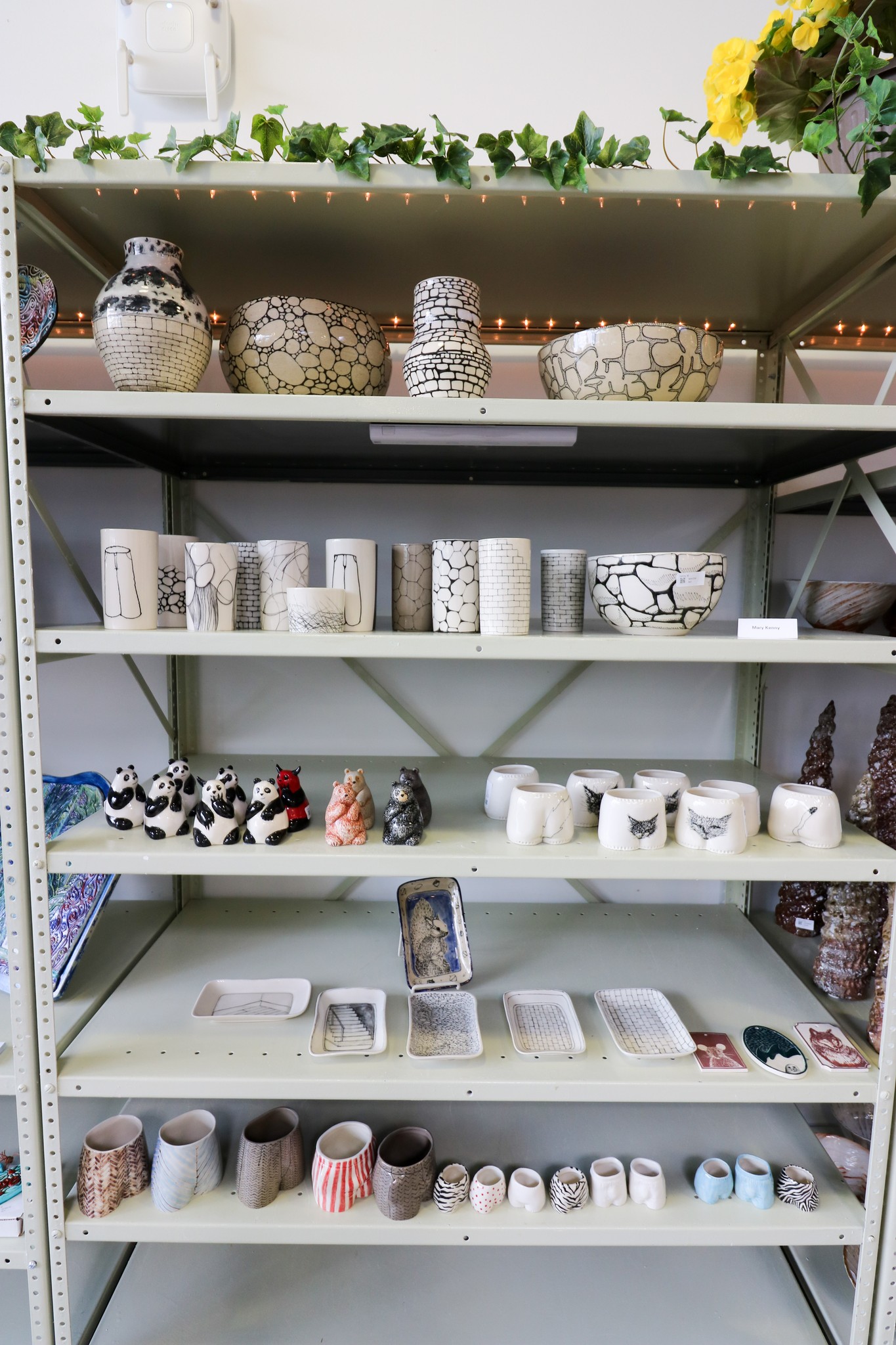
[0,159,896,1345]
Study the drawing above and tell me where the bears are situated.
[343,768,375,830]
[399,767,432,827]
[382,781,424,846]
[324,780,366,846]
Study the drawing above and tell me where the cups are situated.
[484,765,842,854]
[326,538,375,632]
[540,549,587,632]
[159,534,198,628]
[184,542,238,631]
[76,1107,820,1220]
[225,542,260,630]
[99,529,159,629]
[477,538,532,636]
[391,543,431,632]
[430,539,479,632]
[258,539,308,631]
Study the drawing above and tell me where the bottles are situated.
[90,236,212,392]
[404,275,490,398]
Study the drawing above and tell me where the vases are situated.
[90,240,215,394]
[401,275,492,397]
[809,58,896,176]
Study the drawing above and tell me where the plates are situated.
[689,1032,746,1072]
[743,1026,807,1079]
[190,978,310,1023]
[309,987,388,1056]
[502,990,587,1056]
[795,1020,872,1072]
[397,877,473,992]
[406,992,485,1059]
[594,987,698,1058]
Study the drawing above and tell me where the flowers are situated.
[704,0,896,147]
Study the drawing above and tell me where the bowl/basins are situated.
[785,581,896,633]
[586,551,727,636]
[218,294,392,395]
[813,1102,874,1289]
[287,588,345,634]
[537,322,723,402]
